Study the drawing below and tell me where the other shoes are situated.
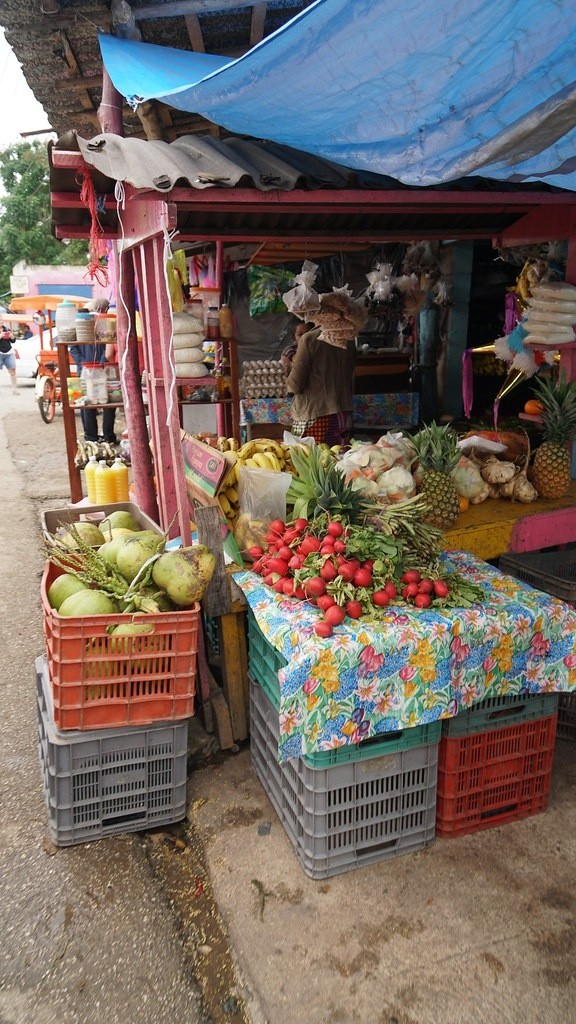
[86,436,106,442]
[110,435,123,445]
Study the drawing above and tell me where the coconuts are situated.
[48,509,216,699]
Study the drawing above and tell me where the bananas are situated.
[215,437,311,519]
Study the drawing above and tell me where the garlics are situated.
[470,454,537,505]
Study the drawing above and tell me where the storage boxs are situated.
[33,502,201,848]
[245,547,576,882]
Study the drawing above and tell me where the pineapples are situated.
[278,419,464,530]
[528,368,576,499]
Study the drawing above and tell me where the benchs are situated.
[40,350,82,377]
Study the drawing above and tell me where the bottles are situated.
[206,305,219,339]
[110,458,129,504]
[94,314,117,343]
[56,298,78,341]
[183,298,204,338]
[219,304,233,339]
[95,460,113,507]
[219,357,232,399]
[80,362,109,405]
[107,303,117,315]
[215,370,225,399]
[139,368,150,405]
[75,311,94,342]
[119,434,130,456]
[84,456,100,504]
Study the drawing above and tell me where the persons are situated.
[280,320,353,448]
[0,317,55,395]
[69,298,122,446]
[280,318,316,370]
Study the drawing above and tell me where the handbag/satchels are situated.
[248,240,455,350]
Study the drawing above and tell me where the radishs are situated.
[237,518,484,637]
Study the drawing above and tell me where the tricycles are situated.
[8,295,95,424]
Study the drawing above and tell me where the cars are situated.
[12,327,77,388]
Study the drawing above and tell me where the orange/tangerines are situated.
[525,400,545,415]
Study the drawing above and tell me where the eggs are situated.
[241,360,288,395]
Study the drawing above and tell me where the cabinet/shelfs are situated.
[55,336,242,505]
